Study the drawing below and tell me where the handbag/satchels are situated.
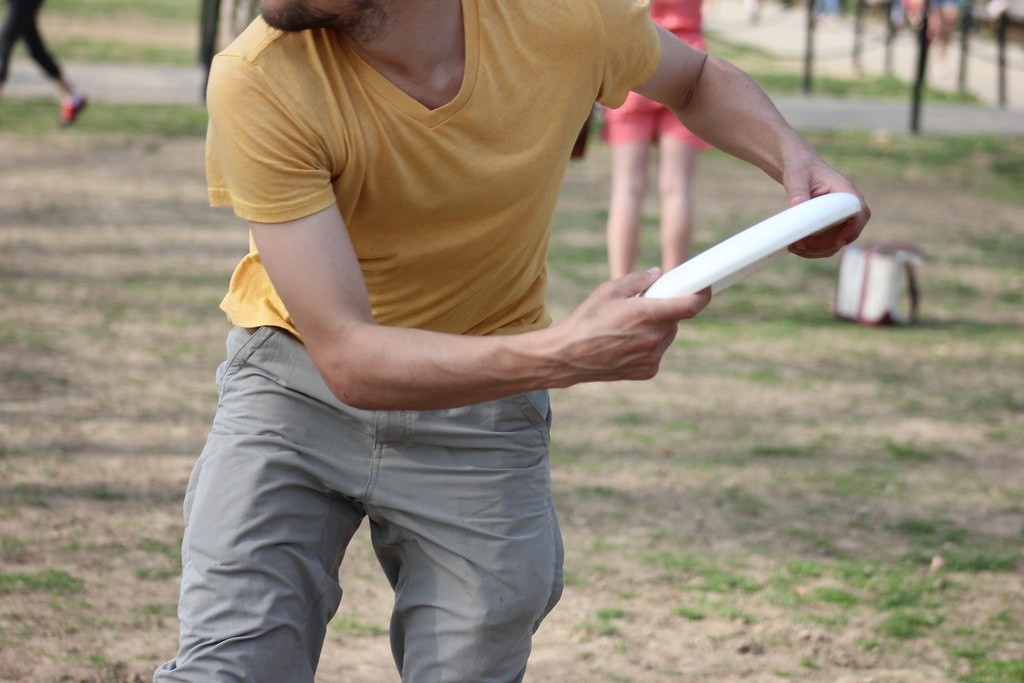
[834,241,928,324]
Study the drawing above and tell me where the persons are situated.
[151,0,871,682]
[0,0,87,127]
[603,0,710,279]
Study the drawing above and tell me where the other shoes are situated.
[61,97,85,125]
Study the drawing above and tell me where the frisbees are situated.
[628,191,864,296]
